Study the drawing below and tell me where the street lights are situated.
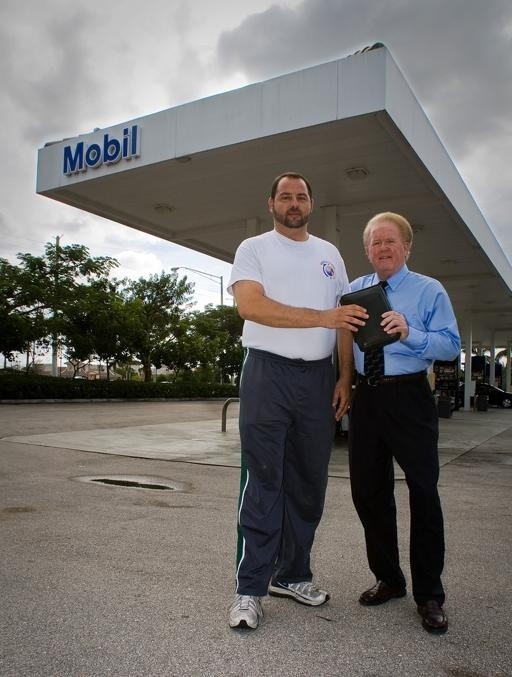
[169,266,225,384]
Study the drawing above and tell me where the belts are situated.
[354,369,427,388]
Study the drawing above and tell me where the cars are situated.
[459,382,512,409]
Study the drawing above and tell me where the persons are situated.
[349,212,461,635]
[229,173,369,630]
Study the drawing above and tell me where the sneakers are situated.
[266,578,331,607]
[227,594,264,630]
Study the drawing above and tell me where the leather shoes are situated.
[416,601,448,634]
[359,580,408,606]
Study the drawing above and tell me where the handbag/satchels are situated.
[340,284,400,352]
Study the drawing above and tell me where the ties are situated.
[363,281,388,390]
